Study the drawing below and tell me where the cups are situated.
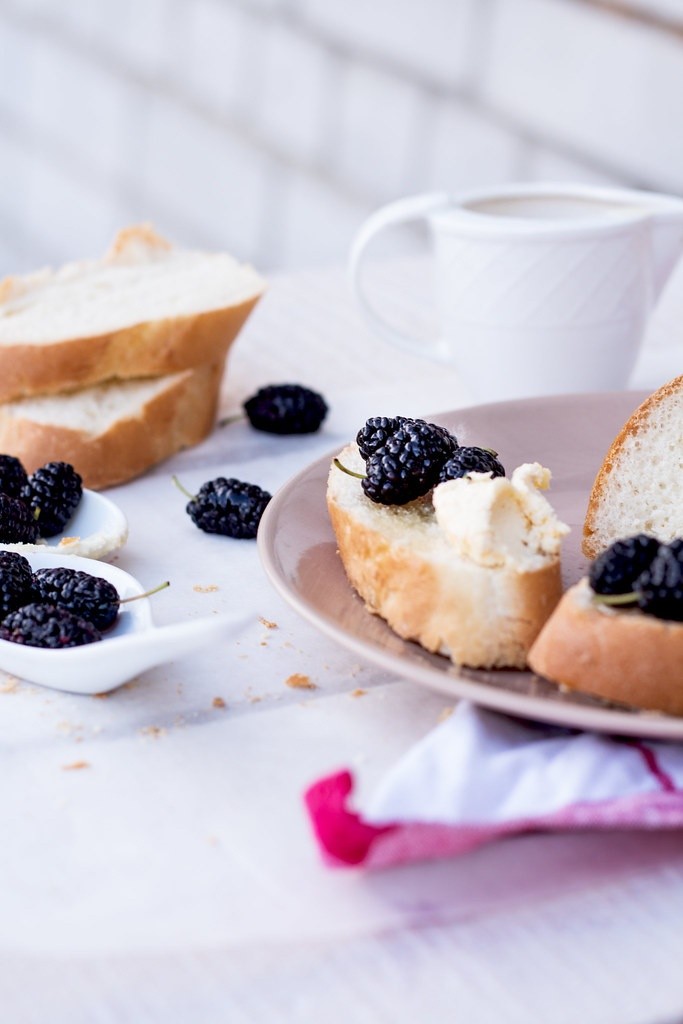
[347,184,683,397]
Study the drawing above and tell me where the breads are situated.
[527,375,683,723]
[0,225,264,492]
[326,439,567,671]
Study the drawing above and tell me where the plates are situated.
[1,488,127,561]
[0,552,163,699]
[257,389,683,742]
